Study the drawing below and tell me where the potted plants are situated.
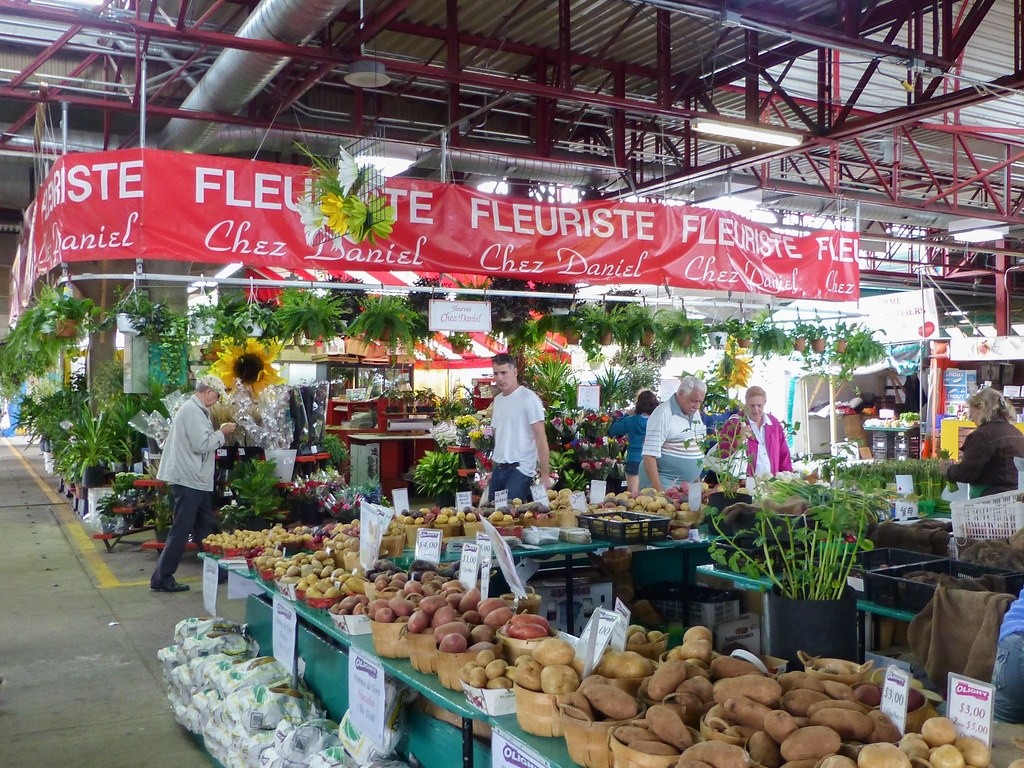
[0,280,473,542]
[488,299,887,361]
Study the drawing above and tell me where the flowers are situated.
[702,398,741,450]
[274,466,344,524]
[206,337,287,398]
[292,137,396,246]
[453,406,630,491]
[314,482,383,522]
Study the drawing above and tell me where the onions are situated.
[762,471,830,488]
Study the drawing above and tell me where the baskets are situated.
[573,509,673,546]
[949,489,1024,543]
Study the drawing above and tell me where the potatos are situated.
[546,479,747,539]
[458,625,1024,768]
[202,498,550,654]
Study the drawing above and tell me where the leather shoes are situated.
[149,581,190,593]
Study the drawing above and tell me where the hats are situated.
[195,375,230,401]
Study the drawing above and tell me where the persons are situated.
[608,391,659,493]
[718,386,793,480]
[638,376,709,492]
[992,585,1024,724]
[940,387,1024,499]
[489,353,550,502]
[150,375,237,591]
[702,387,740,484]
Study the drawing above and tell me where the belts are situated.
[498,462,520,471]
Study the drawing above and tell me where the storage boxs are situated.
[202,491,1024,768]
[873,428,920,460]
[943,370,979,416]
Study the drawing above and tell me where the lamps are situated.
[688,118,804,147]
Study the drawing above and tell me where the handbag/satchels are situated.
[704,414,750,480]
